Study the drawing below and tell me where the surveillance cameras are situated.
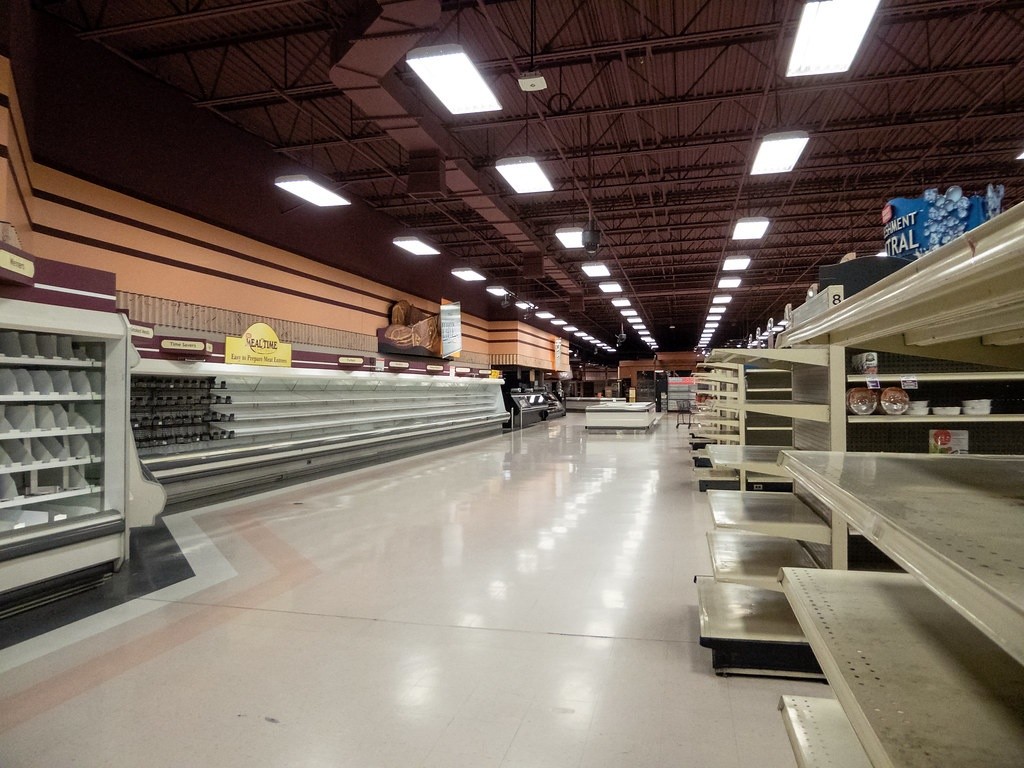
[619,334,626,343]
[582,231,600,254]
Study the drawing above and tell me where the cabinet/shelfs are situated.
[0,201,1024,768]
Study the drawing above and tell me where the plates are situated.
[846,387,909,415]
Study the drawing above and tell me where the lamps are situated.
[749,130,810,175]
[486,286,508,296]
[392,236,441,255]
[406,44,504,114]
[494,156,555,194]
[611,297,631,307]
[451,267,486,281]
[515,301,539,309]
[732,217,769,240]
[598,281,622,292]
[713,294,732,304]
[722,255,751,271]
[718,276,741,288]
[274,174,351,207]
[555,227,585,248]
[581,262,611,277]
[535,305,790,352]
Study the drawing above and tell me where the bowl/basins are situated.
[962,399,992,414]
[905,401,929,415]
[932,407,961,414]
